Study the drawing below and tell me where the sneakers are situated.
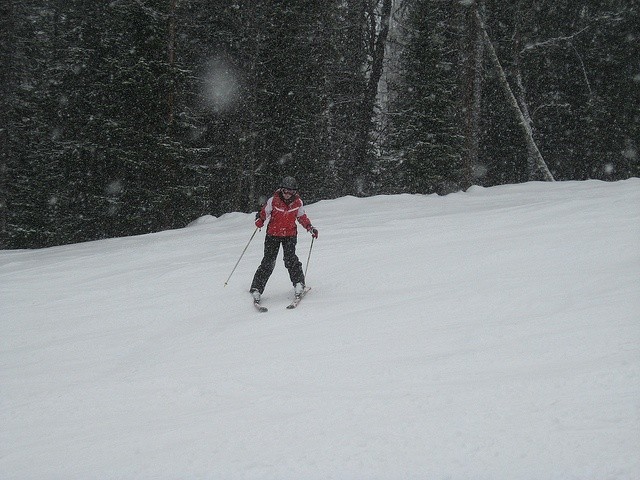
[294,281,305,298]
[249,289,261,303]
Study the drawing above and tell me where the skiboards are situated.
[253,286,311,312]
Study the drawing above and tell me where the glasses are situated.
[281,187,296,196]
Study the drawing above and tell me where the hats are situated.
[279,176,298,190]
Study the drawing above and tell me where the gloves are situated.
[308,224,317,240]
[254,217,263,228]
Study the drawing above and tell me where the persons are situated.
[250,176,319,301]
